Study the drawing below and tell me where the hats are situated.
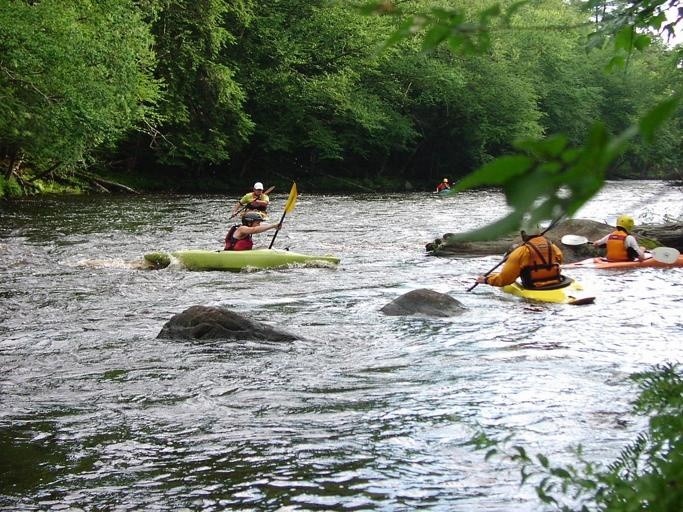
[254,181,264,191]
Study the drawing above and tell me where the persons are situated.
[474,218,563,288]
[436,178,450,194]
[223,210,282,251]
[231,182,270,225]
[591,215,654,262]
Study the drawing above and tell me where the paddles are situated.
[231,185,276,220]
[561,234,680,264]
[269,183,298,250]
[468,210,569,292]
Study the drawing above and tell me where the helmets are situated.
[443,178,449,183]
[242,210,268,225]
[519,216,540,241]
[615,214,634,234]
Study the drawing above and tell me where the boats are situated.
[145,249,340,273]
[438,188,451,196]
[502,275,596,304]
[561,249,683,270]
